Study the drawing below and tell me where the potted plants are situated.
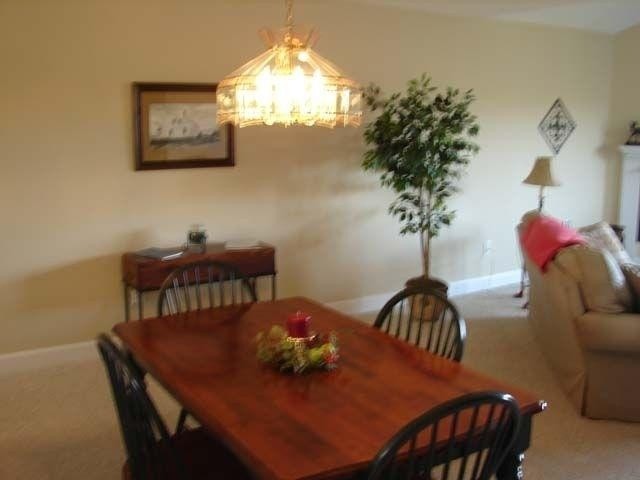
[358,73,482,321]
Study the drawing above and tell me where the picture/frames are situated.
[537,97,576,156]
[132,80,236,172]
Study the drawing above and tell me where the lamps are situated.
[521,156,562,211]
[216,0,364,130]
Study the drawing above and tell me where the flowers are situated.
[253,322,340,376]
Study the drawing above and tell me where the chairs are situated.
[94,330,253,479]
[373,286,467,364]
[156,259,259,319]
[366,389,520,479]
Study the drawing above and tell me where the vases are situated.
[280,329,321,372]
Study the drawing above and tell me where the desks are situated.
[122,238,280,323]
[113,296,548,480]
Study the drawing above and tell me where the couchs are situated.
[516,210,640,422]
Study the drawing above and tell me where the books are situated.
[134,247,183,260]
[225,241,263,249]
